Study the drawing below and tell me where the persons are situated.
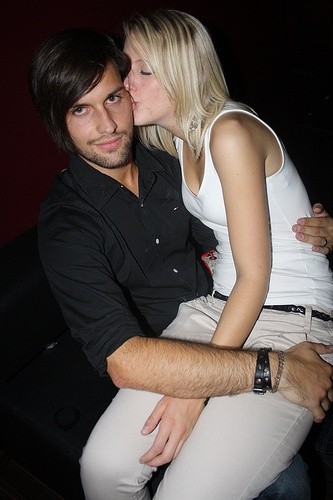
[27,27,333,499]
[80,9,333,500]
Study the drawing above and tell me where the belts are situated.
[209,288,333,321]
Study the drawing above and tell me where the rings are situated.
[320,236,327,248]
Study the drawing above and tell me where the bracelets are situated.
[253,348,272,395]
[276,350,285,395]
